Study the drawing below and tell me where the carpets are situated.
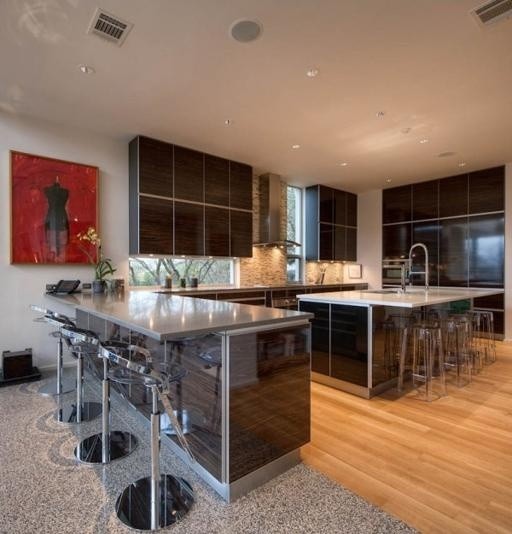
[0,365,418,534]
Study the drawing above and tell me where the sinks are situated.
[358,289,414,295]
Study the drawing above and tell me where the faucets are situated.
[408,243,429,295]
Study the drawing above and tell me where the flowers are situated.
[76,226,117,281]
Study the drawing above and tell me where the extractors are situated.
[251,171,302,251]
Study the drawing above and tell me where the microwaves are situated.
[382,264,412,285]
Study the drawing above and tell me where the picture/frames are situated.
[8,149,101,267]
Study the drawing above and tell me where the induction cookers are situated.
[253,282,303,288]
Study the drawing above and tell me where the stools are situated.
[396,307,497,402]
[28,303,194,533]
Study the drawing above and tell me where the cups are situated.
[165,279,172,288]
[181,278,186,287]
[191,278,198,287]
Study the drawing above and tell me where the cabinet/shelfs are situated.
[305,183,357,263]
[128,134,254,259]
[183,287,355,311]
[380,164,506,342]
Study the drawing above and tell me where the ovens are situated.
[266,289,307,312]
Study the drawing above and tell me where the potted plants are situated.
[92,280,106,293]
